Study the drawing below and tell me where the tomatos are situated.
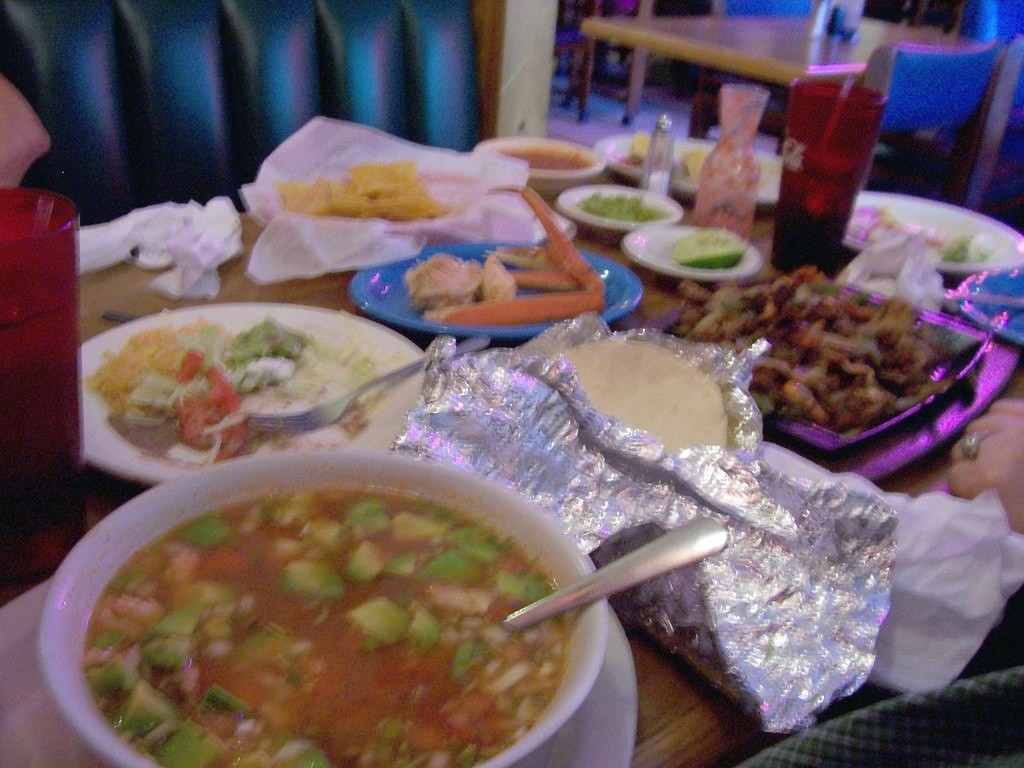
[179,350,244,463]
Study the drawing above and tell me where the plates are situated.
[346,240,645,343]
[79,299,427,486]
[842,189,1024,282]
[954,266,1024,346]
[597,131,783,205]
[622,223,764,281]
[637,277,992,449]
[0,576,638,768]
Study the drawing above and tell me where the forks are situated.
[246,329,491,434]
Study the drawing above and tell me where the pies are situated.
[551,338,728,451]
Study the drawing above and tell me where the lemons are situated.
[673,227,744,268]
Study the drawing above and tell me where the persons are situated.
[947,398,1024,533]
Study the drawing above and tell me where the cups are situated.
[770,77,887,282]
[0,185,84,608]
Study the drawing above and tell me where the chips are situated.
[275,160,454,220]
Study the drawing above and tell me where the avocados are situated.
[95,487,579,767]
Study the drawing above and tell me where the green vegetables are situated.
[123,318,310,416]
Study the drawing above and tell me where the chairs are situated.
[866,33,1023,214]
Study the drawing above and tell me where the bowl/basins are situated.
[556,184,685,245]
[34,444,610,768]
[473,137,607,197]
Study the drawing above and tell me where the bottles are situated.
[690,82,771,240]
[639,113,674,199]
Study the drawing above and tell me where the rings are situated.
[961,431,988,458]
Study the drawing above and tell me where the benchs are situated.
[0,0,557,229]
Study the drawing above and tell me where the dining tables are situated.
[0,191,1024,767]
[577,16,1020,161]
